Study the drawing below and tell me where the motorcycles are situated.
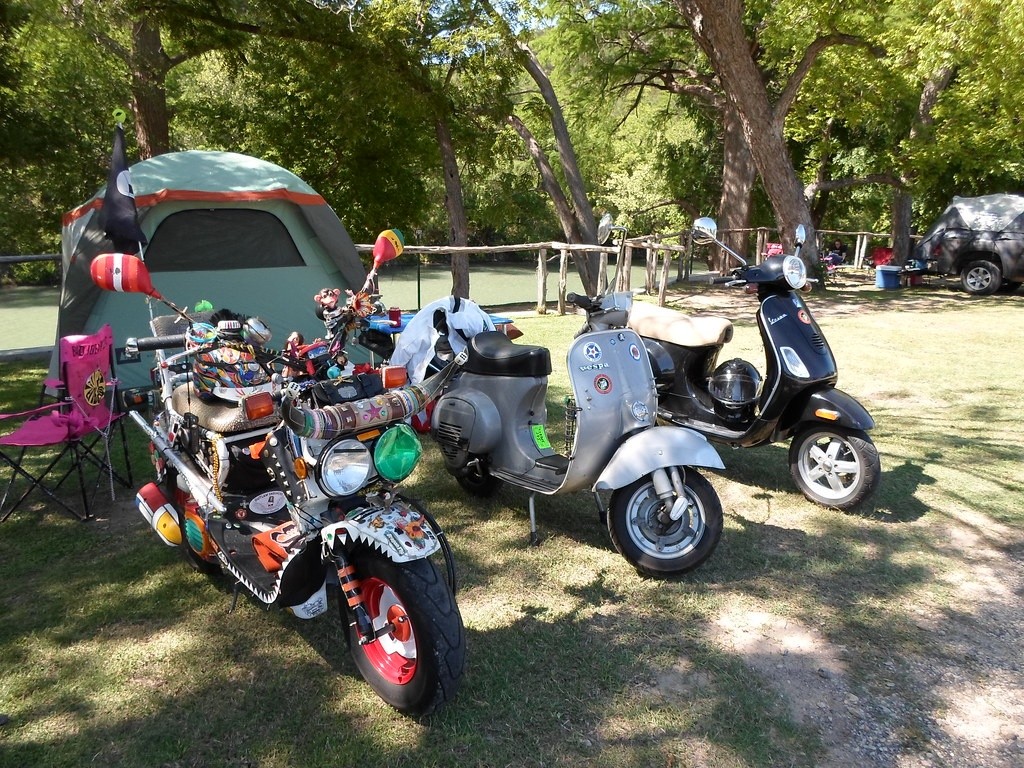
[574,217,881,510]
[89,230,466,715]
[423,213,728,580]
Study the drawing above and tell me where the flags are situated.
[96,125,148,256]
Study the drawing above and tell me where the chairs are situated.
[147,309,317,515]
[0,322,134,523]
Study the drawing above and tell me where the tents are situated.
[44,150,396,402]
[909,193,1024,286]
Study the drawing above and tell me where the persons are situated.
[829,240,847,262]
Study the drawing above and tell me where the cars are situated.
[912,194,1024,296]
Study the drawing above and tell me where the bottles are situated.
[389,307,401,327]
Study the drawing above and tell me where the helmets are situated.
[709,358,764,424]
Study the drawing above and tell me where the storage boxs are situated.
[876,265,902,289]
